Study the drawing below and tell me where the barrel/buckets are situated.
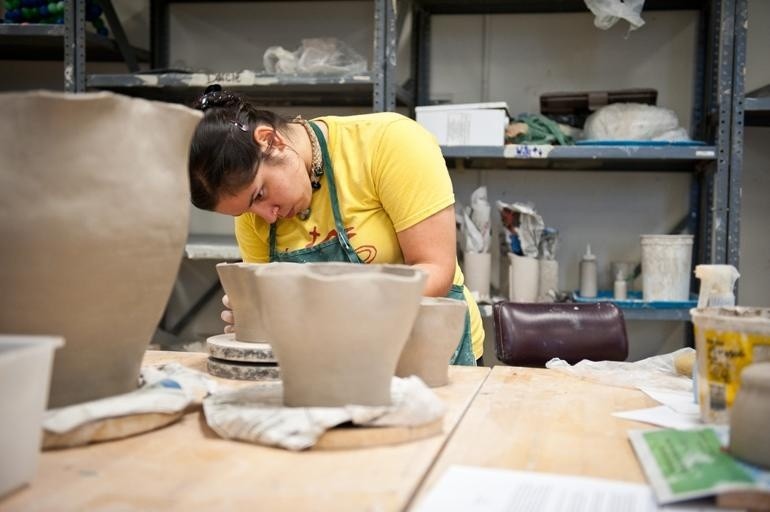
[641,234,694,302]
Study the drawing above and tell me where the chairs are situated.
[491,296,629,371]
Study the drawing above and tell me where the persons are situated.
[187,83,486,367]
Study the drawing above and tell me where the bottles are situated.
[578,239,599,300]
[728,360,769,469]
[613,269,628,300]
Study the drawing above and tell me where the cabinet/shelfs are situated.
[70,0,386,281]
[727,1,770,308]
[0,0,77,96]
[379,0,734,361]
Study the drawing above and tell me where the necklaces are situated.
[291,113,325,193]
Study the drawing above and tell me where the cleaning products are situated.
[695,264,741,308]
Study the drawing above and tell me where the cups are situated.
[538,257,560,303]
[255,260,428,409]
[214,260,272,347]
[396,293,469,389]
[0,90,208,408]
[461,250,491,297]
[507,251,540,305]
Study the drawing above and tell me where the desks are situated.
[405,363,768,511]
[0,331,492,510]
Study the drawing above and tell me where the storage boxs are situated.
[410,95,512,150]
